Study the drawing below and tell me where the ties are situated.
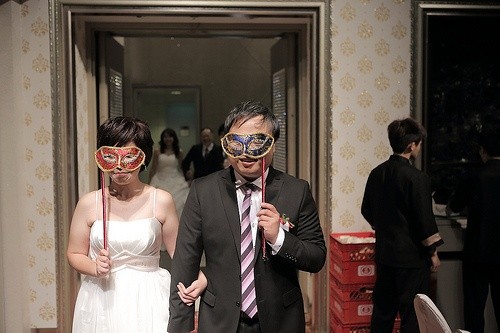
[204,149,209,157]
[239,182,258,318]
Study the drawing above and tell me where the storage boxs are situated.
[329,232,401,333]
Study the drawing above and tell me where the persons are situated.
[362,119,444,333]
[67,117,207,333]
[148,128,190,251]
[167,103,326,333]
[447,130,500,333]
[182,127,224,186]
